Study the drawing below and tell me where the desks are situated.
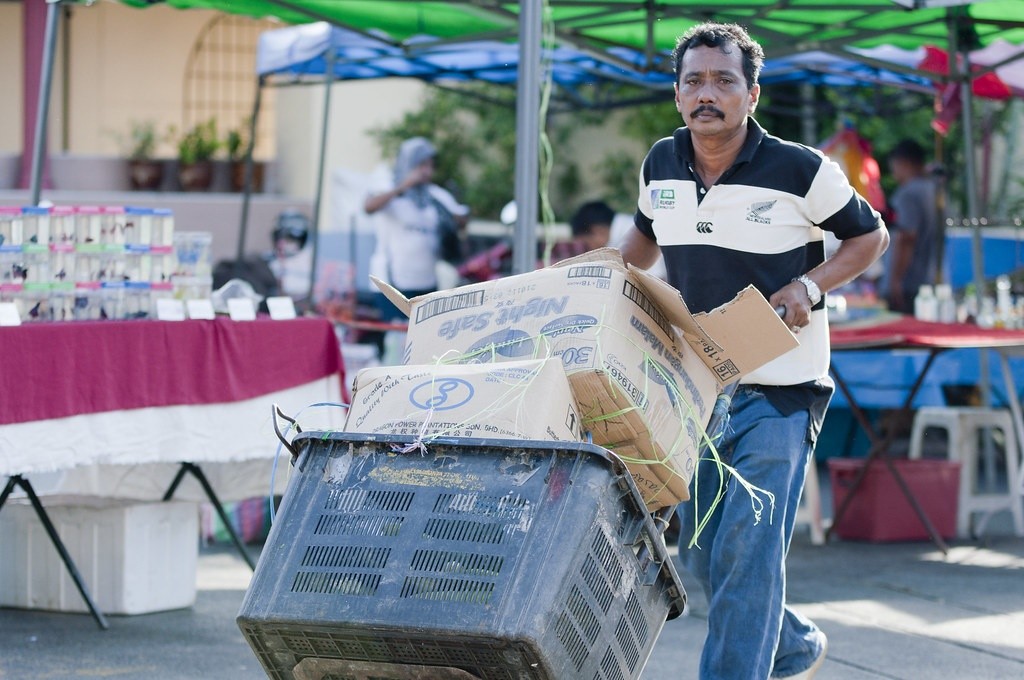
[0,317,350,634]
[823,321,1023,554]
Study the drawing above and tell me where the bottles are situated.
[964,284,977,324]
[915,285,955,323]
[978,275,1024,329]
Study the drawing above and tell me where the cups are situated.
[1,206,175,322]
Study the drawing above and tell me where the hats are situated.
[272,213,309,249]
[878,138,926,166]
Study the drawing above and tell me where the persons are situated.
[618,23,889,680]
[568,199,665,281]
[358,137,470,363]
[215,210,312,308]
[883,139,939,434]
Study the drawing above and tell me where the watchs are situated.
[798,276,821,308]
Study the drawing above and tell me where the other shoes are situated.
[770,633,827,680]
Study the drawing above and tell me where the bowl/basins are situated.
[175,232,211,275]
[173,278,212,319]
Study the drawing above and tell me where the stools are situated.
[909,405,1021,541]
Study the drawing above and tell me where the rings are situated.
[792,326,801,332]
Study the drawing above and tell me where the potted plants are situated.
[174,124,223,192]
[226,119,267,194]
[126,125,163,189]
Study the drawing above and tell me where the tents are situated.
[27,0,1024,490]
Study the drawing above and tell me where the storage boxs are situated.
[236,247,801,678]
[0,492,198,616]
[825,454,960,544]
